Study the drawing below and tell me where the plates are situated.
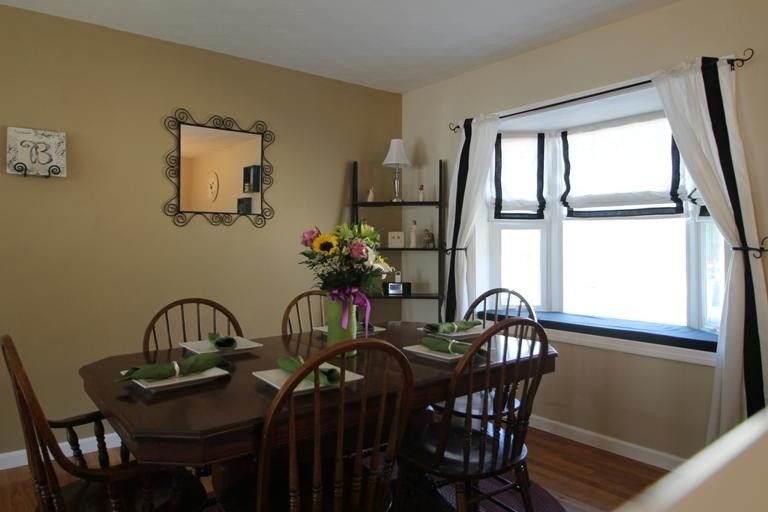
[402,339,475,363]
[252,360,366,398]
[119,366,232,393]
[415,324,487,341]
[178,336,264,358]
[313,324,387,338]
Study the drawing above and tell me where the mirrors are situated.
[162,107,274,230]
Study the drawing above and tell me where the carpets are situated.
[361,451,565,512]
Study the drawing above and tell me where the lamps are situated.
[381,139,412,202]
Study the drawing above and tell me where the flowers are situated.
[296,216,396,298]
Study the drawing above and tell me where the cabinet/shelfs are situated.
[350,159,444,322]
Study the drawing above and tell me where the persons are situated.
[408,219,418,248]
[423,227,433,248]
